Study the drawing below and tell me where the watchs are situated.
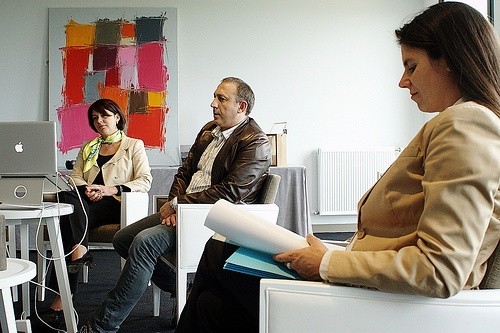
[169,200,174,209]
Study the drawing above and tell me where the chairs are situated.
[37,192,151,287]
[259,239,500,333]
[153,174,282,325]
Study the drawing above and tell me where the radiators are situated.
[311,147,402,216]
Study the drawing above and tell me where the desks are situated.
[0,202,78,333]
[149,167,312,238]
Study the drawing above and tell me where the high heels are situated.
[66,250,94,273]
[33,306,66,324]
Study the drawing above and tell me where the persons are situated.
[75,77,271,333]
[48,99,153,315]
[174,1,500,333]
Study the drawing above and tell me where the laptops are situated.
[0,122,57,176]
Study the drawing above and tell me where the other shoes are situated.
[77,319,99,333]
